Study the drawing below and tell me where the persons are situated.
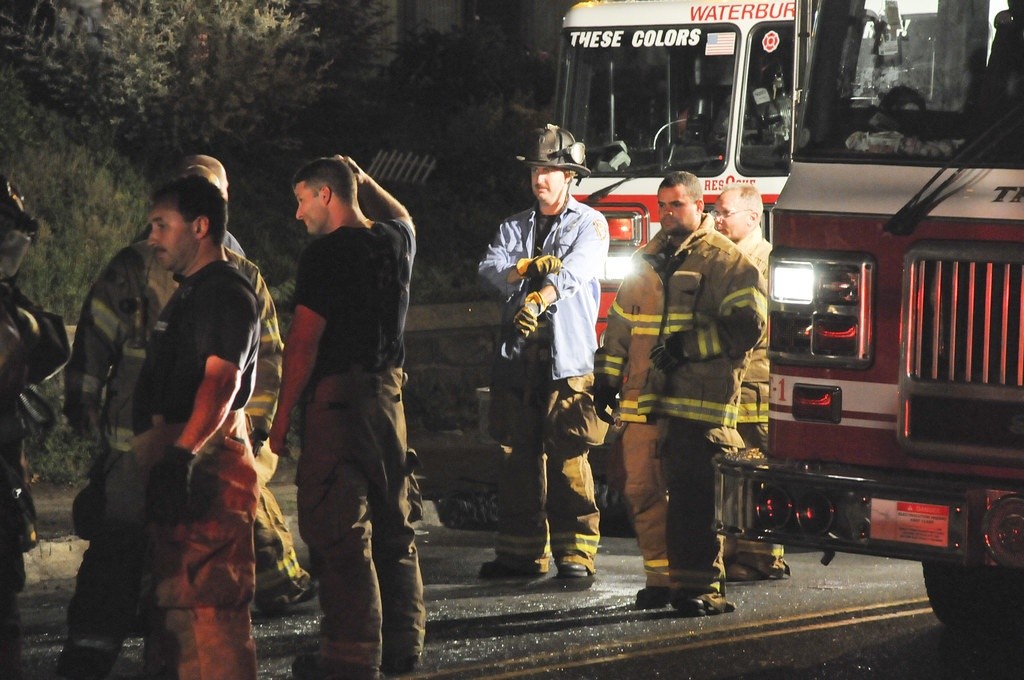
[594,170,790,615]
[0,154,320,678]
[474,124,610,581]
[129,174,261,679]
[269,154,427,680]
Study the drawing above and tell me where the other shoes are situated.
[725,561,767,581]
[291,654,380,680]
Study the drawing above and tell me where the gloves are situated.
[594,385,618,425]
[515,255,561,277]
[512,291,547,337]
[148,445,196,523]
[647,345,679,373]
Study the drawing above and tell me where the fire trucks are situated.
[708,1,1024,667]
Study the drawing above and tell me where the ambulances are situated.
[549,1,803,536]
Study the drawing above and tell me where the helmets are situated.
[514,128,591,178]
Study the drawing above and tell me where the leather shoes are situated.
[635,586,681,609]
[557,562,587,578]
[679,599,708,617]
[480,561,523,576]
